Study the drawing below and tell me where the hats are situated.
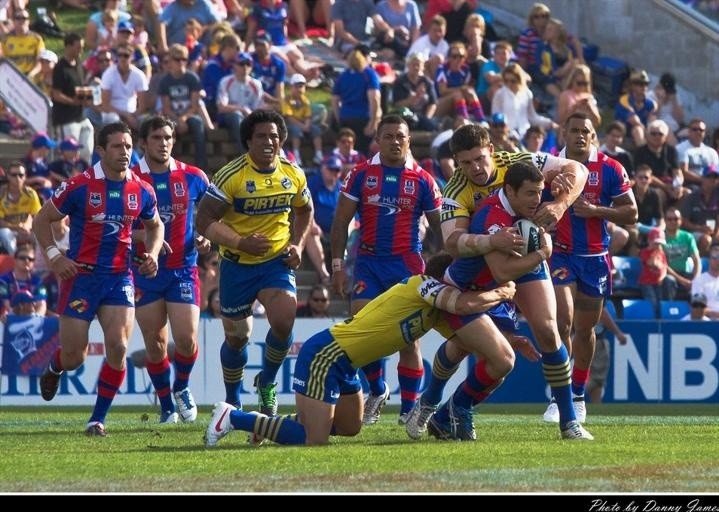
[14,10,31,20]
[252,30,272,44]
[59,136,85,151]
[38,50,59,64]
[118,21,136,34]
[691,292,707,305]
[659,73,678,94]
[289,73,307,85]
[10,289,42,308]
[703,162,719,177]
[235,52,253,63]
[647,227,667,245]
[324,156,343,170]
[30,132,56,150]
[627,70,652,85]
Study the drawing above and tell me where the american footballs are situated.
[512,219,540,256]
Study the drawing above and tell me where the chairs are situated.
[596,249,717,322]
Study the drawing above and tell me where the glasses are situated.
[116,53,131,59]
[451,54,465,59]
[576,80,588,86]
[692,303,706,308]
[173,57,189,62]
[17,256,35,262]
[635,175,649,180]
[649,132,664,137]
[313,297,328,302]
[692,128,705,132]
[533,14,547,19]
[666,216,680,221]
[9,173,25,178]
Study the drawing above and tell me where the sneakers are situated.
[160,402,178,427]
[570,396,587,424]
[543,395,563,424]
[202,401,238,448]
[360,380,391,426]
[39,345,64,402]
[397,392,477,442]
[84,420,109,439]
[173,382,198,424]
[247,410,269,450]
[560,420,595,441]
[253,370,278,417]
